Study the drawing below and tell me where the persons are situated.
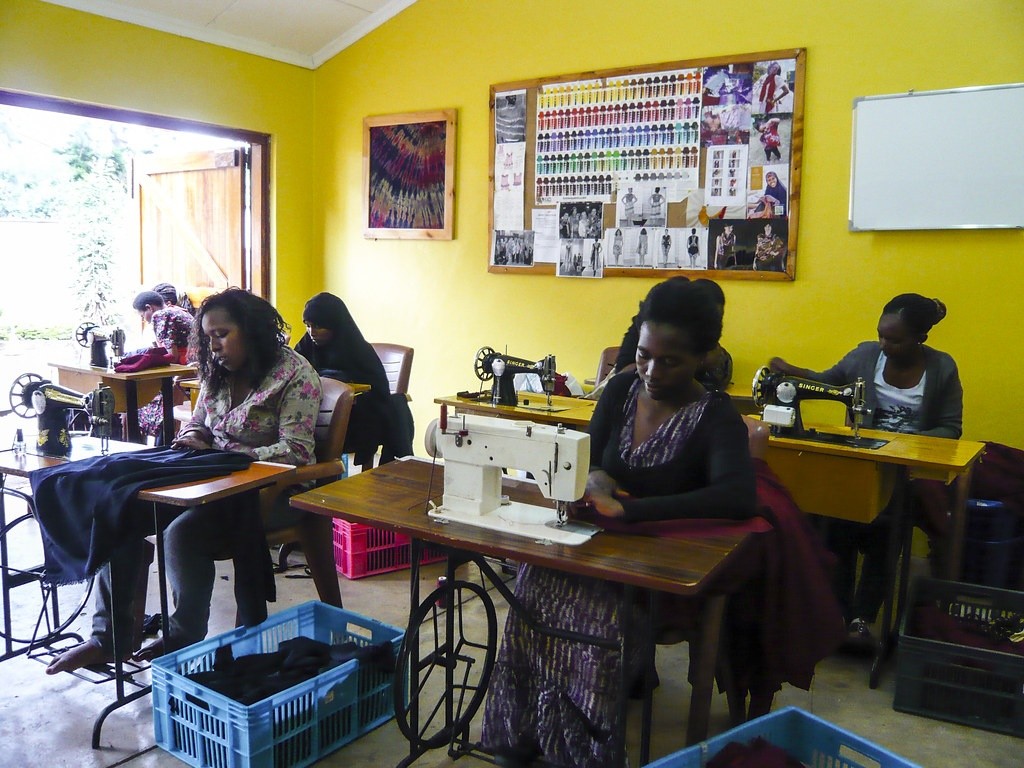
[46,289,322,674]
[749,171,787,218]
[753,62,790,115]
[760,118,781,161]
[753,221,786,272]
[559,207,603,276]
[480,276,756,768]
[613,228,700,268]
[622,188,666,227]
[295,292,390,454]
[122,283,204,446]
[714,220,737,270]
[768,293,963,641]
[494,236,533,266]
[713,151,736,196]
[719,64,742,104]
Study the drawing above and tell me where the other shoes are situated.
[847,622,871,641]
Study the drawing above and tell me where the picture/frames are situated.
[361,108,458,242]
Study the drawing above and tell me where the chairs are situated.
[216,377,354,607]
[591,345,622,391]
[369,342,415,398]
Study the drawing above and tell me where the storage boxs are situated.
[893,574,1024,737]
[150,599,410,768]
[331,517,447,579]
[643,706,921,768]
